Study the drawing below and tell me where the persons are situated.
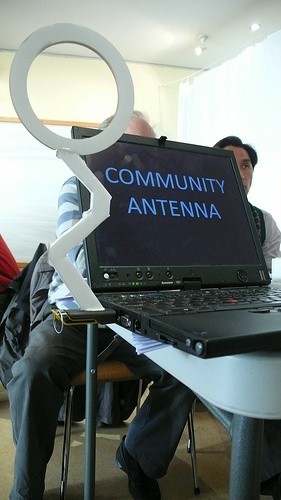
[5,111,195,499]
[1,230,154,430]
[214,137,281,500]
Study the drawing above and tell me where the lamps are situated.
[195,34,209,55]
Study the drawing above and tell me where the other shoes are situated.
[261,474,281,498]
[115,435,161,499]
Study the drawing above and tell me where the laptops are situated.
[71,127,281,359]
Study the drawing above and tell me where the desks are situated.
[84,321,281,500]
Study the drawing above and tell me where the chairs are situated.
[30,251,201,500]
